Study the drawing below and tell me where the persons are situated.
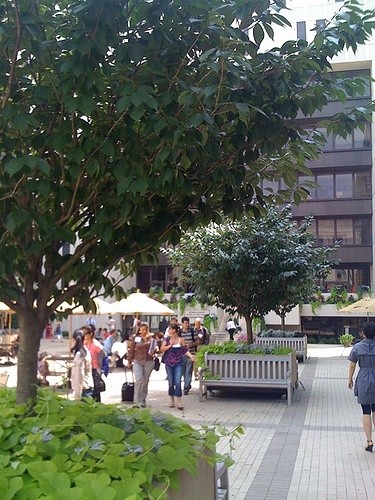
[347,322,375,452]
[179,317,200,395]
[226,318,242,341]
[128,322,159,409]
[70,329,94,404]
[159,323,198,410]
[191,317,210,381]
[202,309,218,333]
[85,314,178,374]
[55,318,63,343]
[80,324,105,403]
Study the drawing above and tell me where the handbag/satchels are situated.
[203,328,209,345]
[154,357,160,371]
[92,368,105,393]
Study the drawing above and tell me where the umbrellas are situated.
[97,289,175,333]
[47,294,110,363]
[0,301,37,366]
[339,296,375,322]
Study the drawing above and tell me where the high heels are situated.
[365,443,374,452]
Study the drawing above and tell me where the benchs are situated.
[206,427,229,500]
[256,335,306,364]
[197,351,293,406]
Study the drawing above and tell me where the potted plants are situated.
[338,333,355,347]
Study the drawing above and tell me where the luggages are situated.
[121,366,135,402]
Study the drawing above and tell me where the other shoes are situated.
[177,404,184,410]
[169,403,175,408]
[140,399,146,407]
[132,402,139,409]
[184,389,189,395]
